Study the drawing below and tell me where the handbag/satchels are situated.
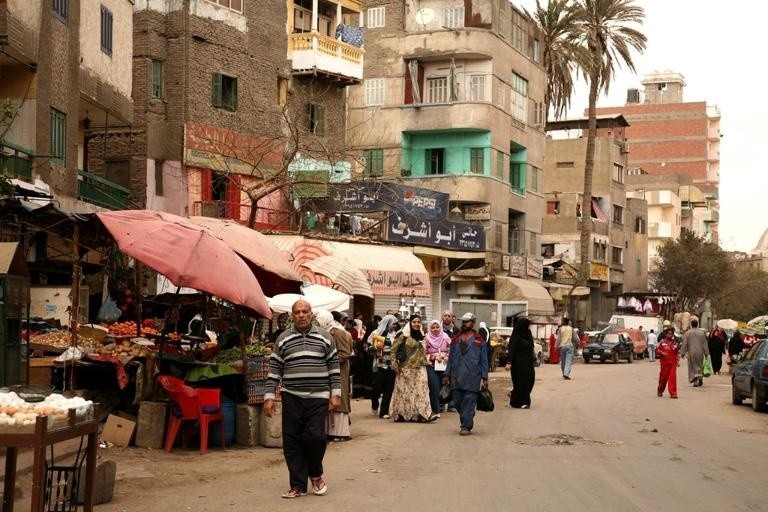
[476,389,494,412]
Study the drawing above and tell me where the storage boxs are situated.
[102,410,138,448]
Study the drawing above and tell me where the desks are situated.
[0,384,101,512]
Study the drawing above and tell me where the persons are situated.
[505,318,537,409]
[274,313,289,344]
[647,319,746,398]
[549,330,560,364]
[554,319,581,379]
[164,308,182,332]
[324,310,491,441]
[263,301,342,498]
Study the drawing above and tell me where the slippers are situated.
[281,487,307,498]
[311,477,327,495]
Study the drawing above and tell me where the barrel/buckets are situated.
[213,400,235,447]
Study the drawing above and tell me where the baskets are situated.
[243,356,282,404]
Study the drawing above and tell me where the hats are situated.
[457,312,478,321]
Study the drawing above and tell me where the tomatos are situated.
[109,320,158,337]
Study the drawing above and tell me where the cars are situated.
[582,332,634,363]
[732,338,768,412]
[611,329,648,360]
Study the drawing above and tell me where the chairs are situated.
[155,374,227,455]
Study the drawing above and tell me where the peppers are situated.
[212,342,266,363]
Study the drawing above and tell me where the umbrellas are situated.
[717,315,768,334]
[96,210,273,402]
[300,255,375,298]
[184,216,305,297]
[268,284,352,316]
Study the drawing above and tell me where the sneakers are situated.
[372,408,378,415]
[693,376,703,387]
[459,427,471,435]
[421,411,440,423]
[382,415,390,419]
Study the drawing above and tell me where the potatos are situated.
[102,340,152,357]
